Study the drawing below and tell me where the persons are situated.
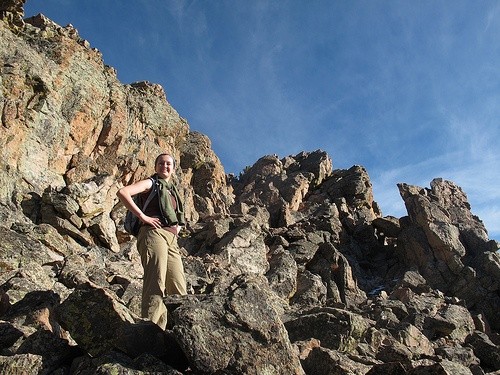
[116,153,188,330]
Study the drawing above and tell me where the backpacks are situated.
[125,177,160,236]
